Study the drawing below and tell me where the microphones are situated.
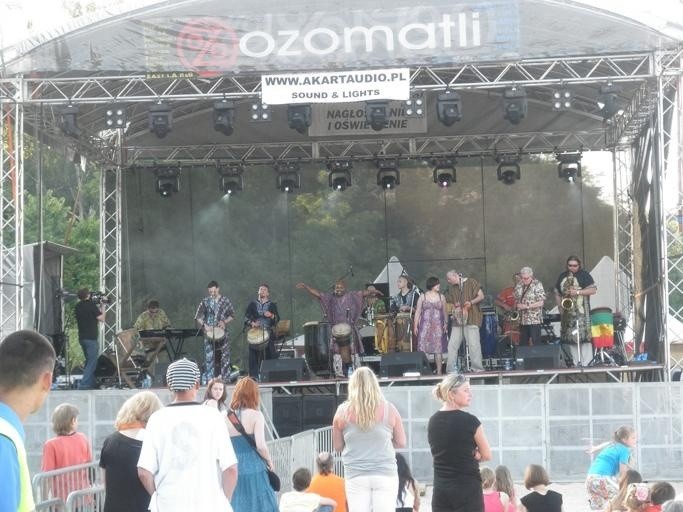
[349,264,355,279]
[257,292,262,303]
[458,272,463,281]
[345,306,350,321]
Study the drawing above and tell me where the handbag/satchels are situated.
[227,410,280,492]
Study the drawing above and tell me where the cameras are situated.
[88,289,115,308]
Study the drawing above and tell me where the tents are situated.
[551,256,683,348]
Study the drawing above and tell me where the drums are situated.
[207,326,224,339]
[332,323,351,363]
[247,328,269,351]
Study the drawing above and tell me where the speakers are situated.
[272,395,303,437]
[96,355,117,388]
[302,394,337,430]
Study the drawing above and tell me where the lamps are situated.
[272,164,301,193]
[551,89,573,112]
[326,162,352,191]
[153,167,181,196]
[436,93,463,127]
[595,84,622,117]
[502,90,528,124]
[105,104,126,128]
[211,101,237,135]
[372,160,401,189]
[364,100,389,131]
[286,104,314,134]
[246,100,272,122]
[493,156,521,184]
[429,159,457,189]
[215,165,243,195]
[555,155,582,184]
[148,104,173,138]
[59,109,81,137]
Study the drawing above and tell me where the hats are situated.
[165,357,200,392]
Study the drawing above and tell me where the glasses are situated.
[449,374,465,391]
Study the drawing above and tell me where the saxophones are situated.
[562,271,573,310]
[509,282,523,324]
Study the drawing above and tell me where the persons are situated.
[194,280,236,385]
[446,270,485,375]
[428,374,492,512]
[585,425,636,512]
[305,452,348,512]
[296,282,384,378]
[333,366,406,512]
[279,468,337,512]
[643,482,676,512]
[201,378,229,412]
[99,391,163,512]
[137,357,238,512]
[513,267,546,345]
[494,466,516,512]
[224,377,280,512]
[625,482,650,512]
[0,331,56,512]
[495,273,522,346]
[363,286,386,313]
[244,284,280,382]
[414,277,449,375]
[395,452,420,512]
[554,255,598,367]
[519,464,563,512]
[662,498,683,512]
[604,470,641,512]
[75,288,108,390]
[133,301,172,358]
[391,276,419,314]
[480,468,509,512]
[39,403,95,512]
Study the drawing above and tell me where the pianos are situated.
[138,327,203,337]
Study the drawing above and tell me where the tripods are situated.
[97,329,137,389]
[55,336,85,390]
